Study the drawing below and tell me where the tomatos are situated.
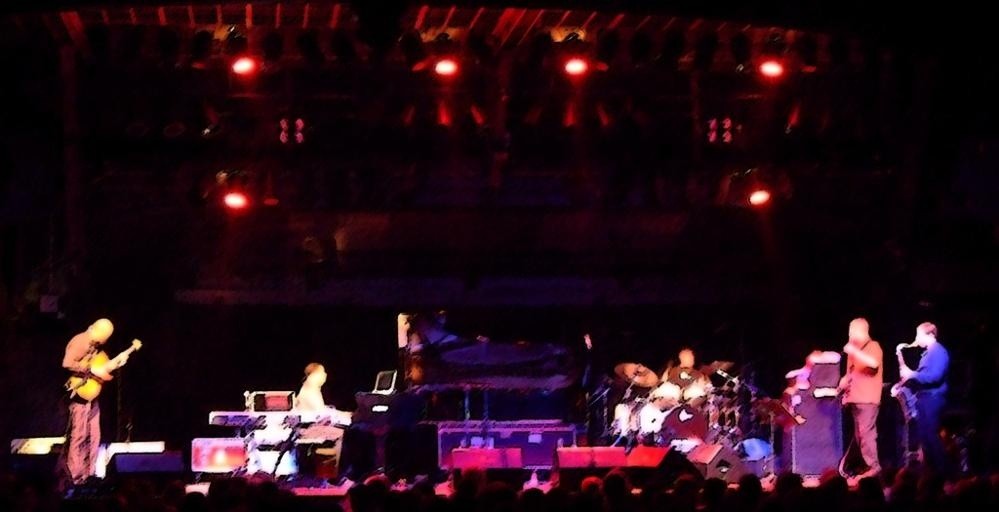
[350,311,589,477]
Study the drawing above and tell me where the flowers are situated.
[715,368,737,382]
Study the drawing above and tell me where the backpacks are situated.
[661,406,707,450]
[630,400,662,432]
[614,398,638,437]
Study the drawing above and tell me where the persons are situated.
[650,349,713,404]
[1,397,999,511]
[61,319,130,482]
[836,318,883,480]
[890,322,950,491]
[293,361,355,479]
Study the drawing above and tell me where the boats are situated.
[551,445,625,491]
[620,444,705,491]
[449,447,523,492]
[781,391,844,475]
[104,452,185,486]
[686,443,750,484]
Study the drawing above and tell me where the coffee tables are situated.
[614,363,659,387]
[698,362,732,373]
